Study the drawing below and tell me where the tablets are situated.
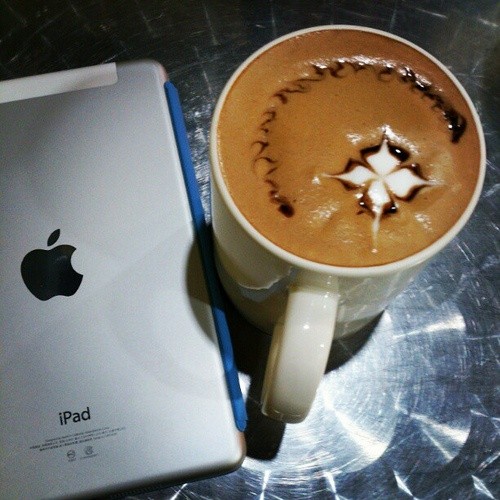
[2,57,247,500]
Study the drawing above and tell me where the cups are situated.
[208,23,487,423]
